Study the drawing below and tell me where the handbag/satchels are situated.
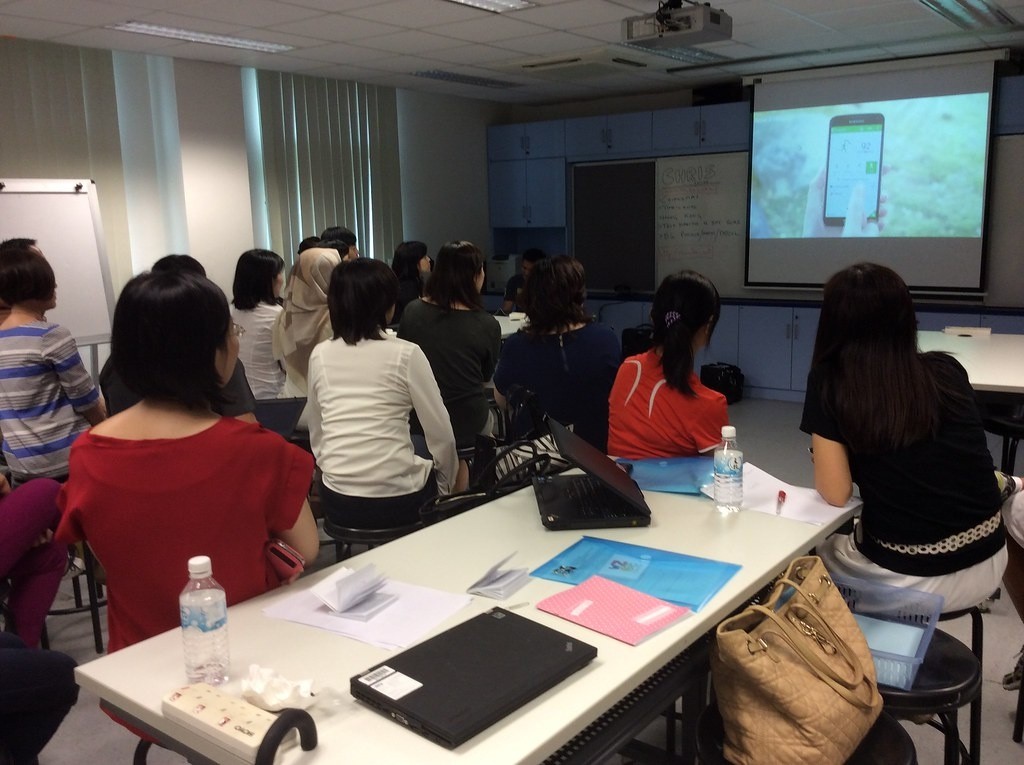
[420,440,552,528]
[700,361,745,405]
[621,322,658,359]
[711,555,883,764]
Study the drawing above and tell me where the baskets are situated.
[774,571,944,691]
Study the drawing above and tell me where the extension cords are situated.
[161,682,299,765]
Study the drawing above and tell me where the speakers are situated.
[622,328,655,362]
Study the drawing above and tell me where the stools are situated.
[693,604,988,765]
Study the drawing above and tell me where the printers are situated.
[485,253,523,293]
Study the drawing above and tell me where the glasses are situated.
[226,322,246,343]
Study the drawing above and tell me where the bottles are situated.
[713,426,744,514]
[179,555,232,687]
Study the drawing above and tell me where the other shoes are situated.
[1002,654,1024,691]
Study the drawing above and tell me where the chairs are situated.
[1,311,1023,764]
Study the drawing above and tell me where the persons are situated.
[54,255,321,750]
[232,225,729,525]
[0,238,107,765]
[802,162,889,236]
[994,470,1024,689]
[801,260,1008,611]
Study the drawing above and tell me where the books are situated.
[536,574,691,646]
[467,551,533,601]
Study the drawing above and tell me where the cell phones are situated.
[823,113,885,226]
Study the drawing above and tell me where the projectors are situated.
[621,4,733,49]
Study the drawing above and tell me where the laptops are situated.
[253,397,307,441]
[531,417,651,531]
[349,606,597,750]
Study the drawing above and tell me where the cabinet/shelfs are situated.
[491,158,565,228]
[652,101,749,157]
[487,121,564,162]
[738,306,821,390]
[567,112,652,162]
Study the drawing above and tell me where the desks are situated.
[75,453,863,765]
[913,330,1023,395]
[490,314,528,345]
